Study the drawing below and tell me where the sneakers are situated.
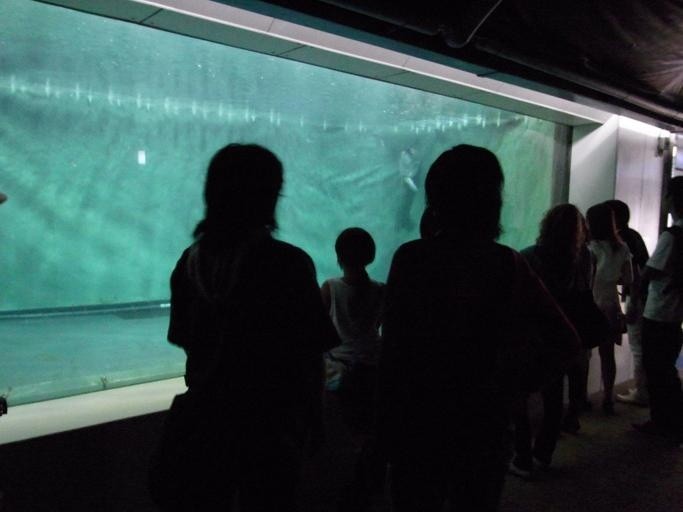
[615,387,650,409]
[508,457,535,480]
[531,449,554,473]
[628,418,681,439]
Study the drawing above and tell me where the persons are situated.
[583,203,634,413]
[632,174,683,451]
[319,227,385,509]
[605,198,650,407]
[377,143,580,511]
[508,204,612,477]
[149,142,343,511]
[562,243,598,440]
